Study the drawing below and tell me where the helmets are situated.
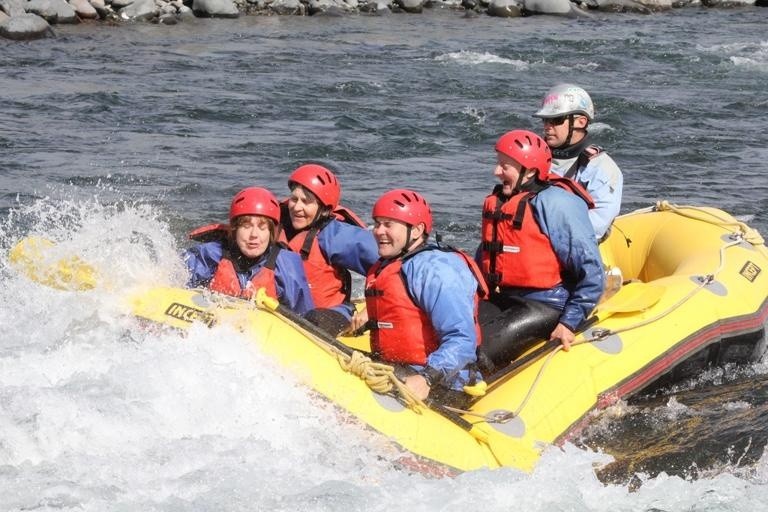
[373,189,432,235]
[495,130,551,182]
[532,84,594,121]
[288,165,340,210]
[231,188,280,223]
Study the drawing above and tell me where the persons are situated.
[474,130,604,377]
[275,165,381,337]
[531,84,623,242]
[179,187,314,316]
[364,189,488,415]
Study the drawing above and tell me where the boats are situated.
[14,203,764,483]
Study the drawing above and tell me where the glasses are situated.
[549,117,570,125]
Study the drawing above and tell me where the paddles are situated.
[255,288,543,471]
[464,282,666,398]
